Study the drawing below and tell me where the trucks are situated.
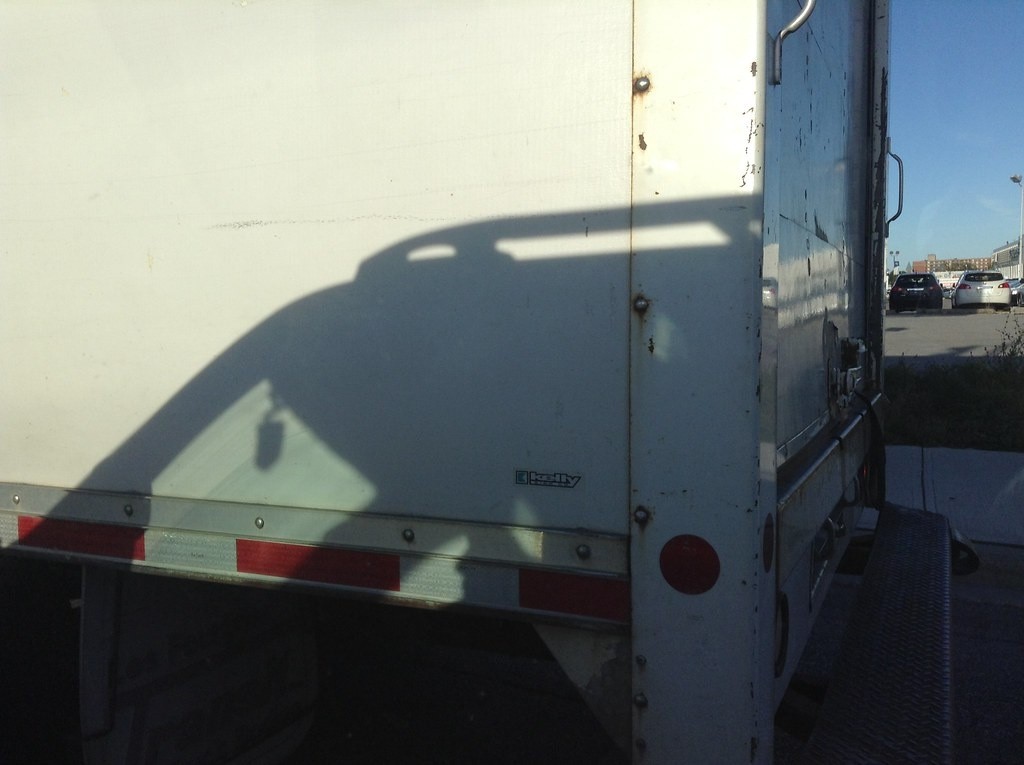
[2,2,905,764]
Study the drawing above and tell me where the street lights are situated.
[890,251,899,261]
[1010,174,1024,279]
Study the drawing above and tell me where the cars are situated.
[888,273,944,313]
[941,287,956,299]
[950,271,1012,310]
[1005,278,1024,306]
